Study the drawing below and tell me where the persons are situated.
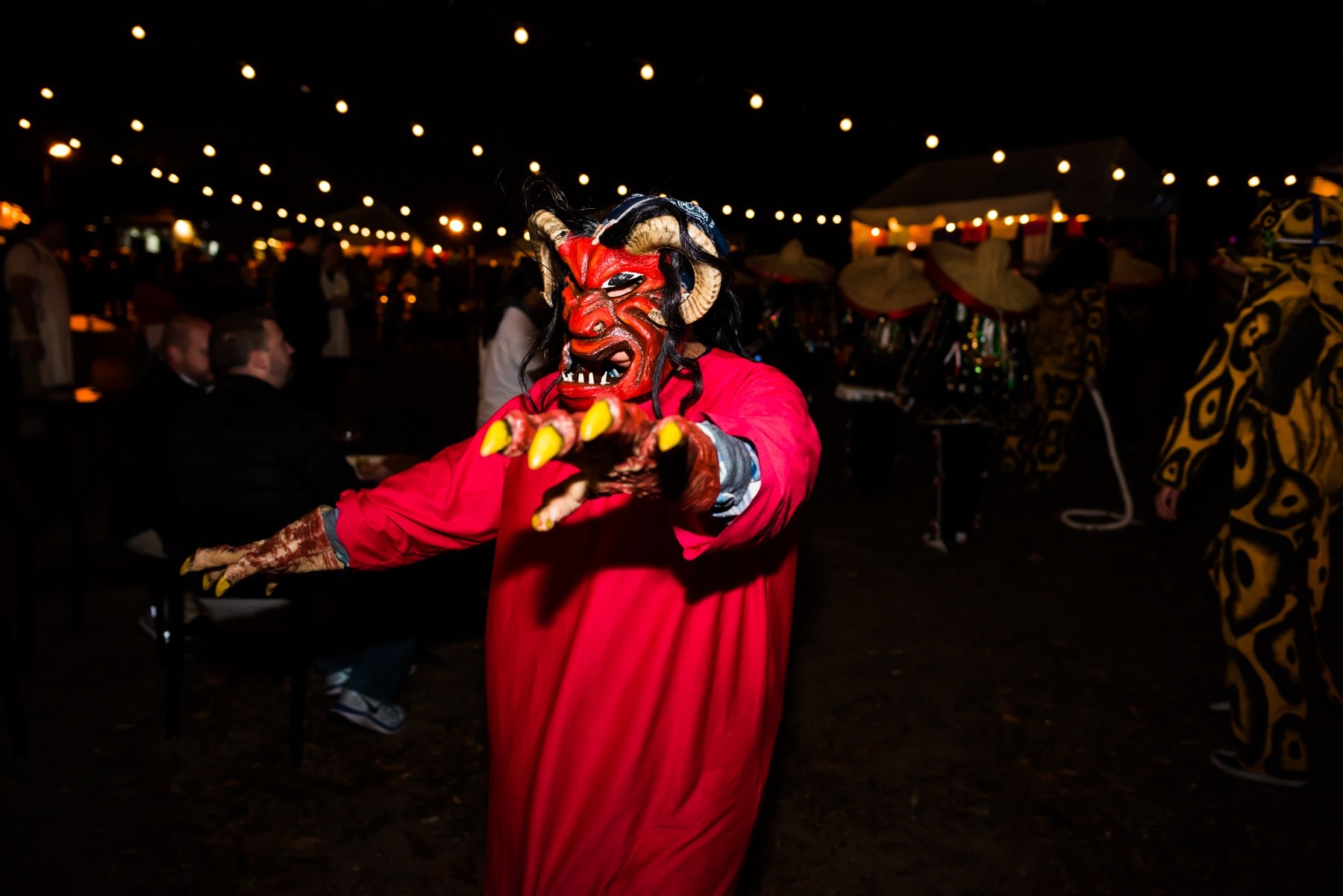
[1155,190,1342,789]
[180,166,823,896]
[160,301,422,736]
[91,308,220,657]
[1,196,1343,559]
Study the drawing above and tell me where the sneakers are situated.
[325,667,356,696]
[331,688,405,734]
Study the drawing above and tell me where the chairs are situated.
[67,438,376,770]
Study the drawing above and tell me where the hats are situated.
[936,239,1040,311]
[747,238,836,279]
[840,249,936,307]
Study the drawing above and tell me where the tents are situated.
[849,133,1168,255]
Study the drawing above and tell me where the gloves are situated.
[480,396,721,534]
[179,505,351,599]
